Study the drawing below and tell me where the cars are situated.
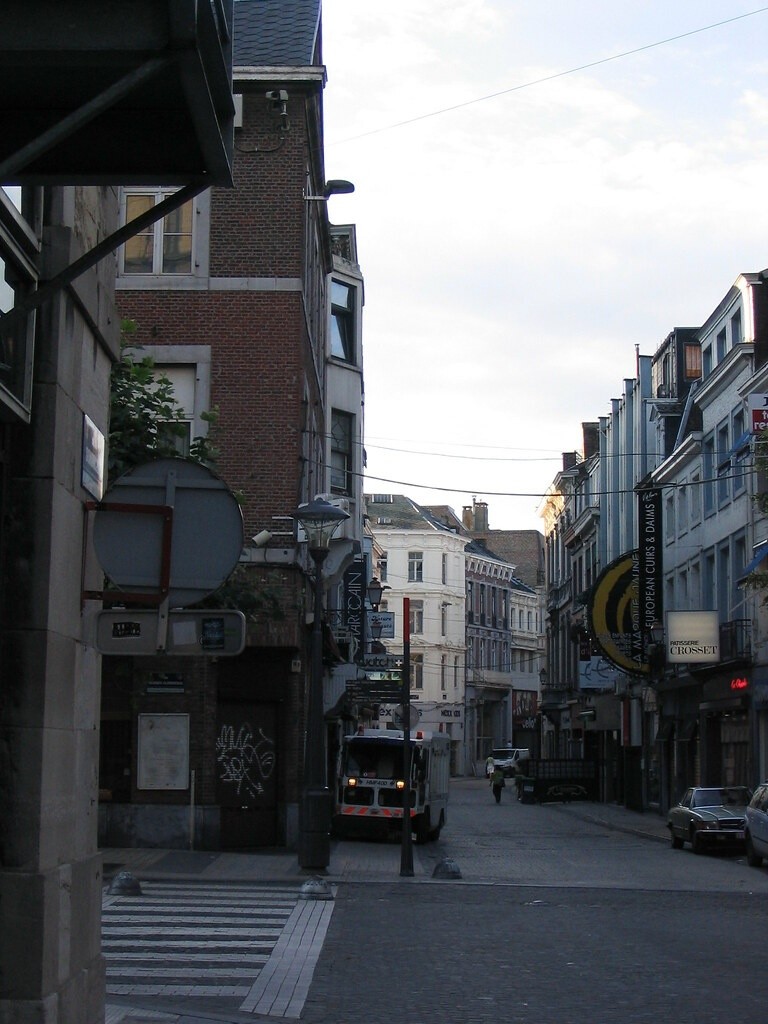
[665,785,754,858]
[742,783,768,869]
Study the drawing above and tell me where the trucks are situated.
[337,726,452,843]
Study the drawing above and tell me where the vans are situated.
[490,747,531,779]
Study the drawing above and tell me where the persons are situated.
[486,754,493,776]
[490,765,505,803]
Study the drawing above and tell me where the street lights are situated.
[285,497,352,876]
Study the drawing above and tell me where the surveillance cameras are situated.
[251,531,272,547]
[266,91,280,100]
